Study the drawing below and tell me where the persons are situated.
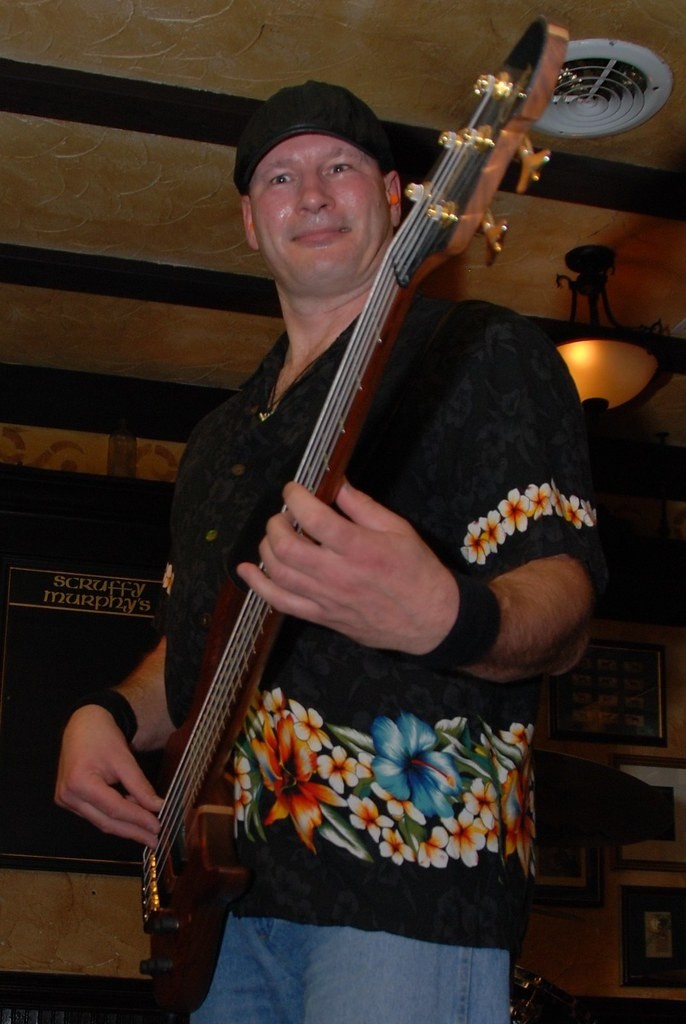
[52,79,597,1024]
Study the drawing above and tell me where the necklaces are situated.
[258,353,323,422]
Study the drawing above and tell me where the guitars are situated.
[131,7,578,1013]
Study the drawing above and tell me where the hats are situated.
[233,79,395,195]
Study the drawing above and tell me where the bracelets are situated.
[60,688,138,745]
[376,572,498,669]
